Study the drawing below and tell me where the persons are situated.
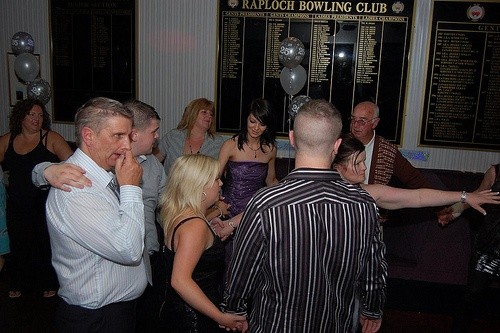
[0,96,500,333]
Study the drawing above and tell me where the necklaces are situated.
[189,141,203,154]
[244,139,265,158]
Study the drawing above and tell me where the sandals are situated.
[44,291,56,297]
[9,290,21,297]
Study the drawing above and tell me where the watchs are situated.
[460,190,467,203]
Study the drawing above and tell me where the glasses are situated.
[350,117,379,125]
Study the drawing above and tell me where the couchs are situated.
[274,156,484,284]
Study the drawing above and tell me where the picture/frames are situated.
[7,52,40,107]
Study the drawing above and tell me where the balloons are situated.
[11,32,53,105]
[277,36,313,121]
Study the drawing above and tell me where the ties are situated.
[107,178,153,286]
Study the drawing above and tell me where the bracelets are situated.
[212,205,223,218]
[228,219,237,229]
